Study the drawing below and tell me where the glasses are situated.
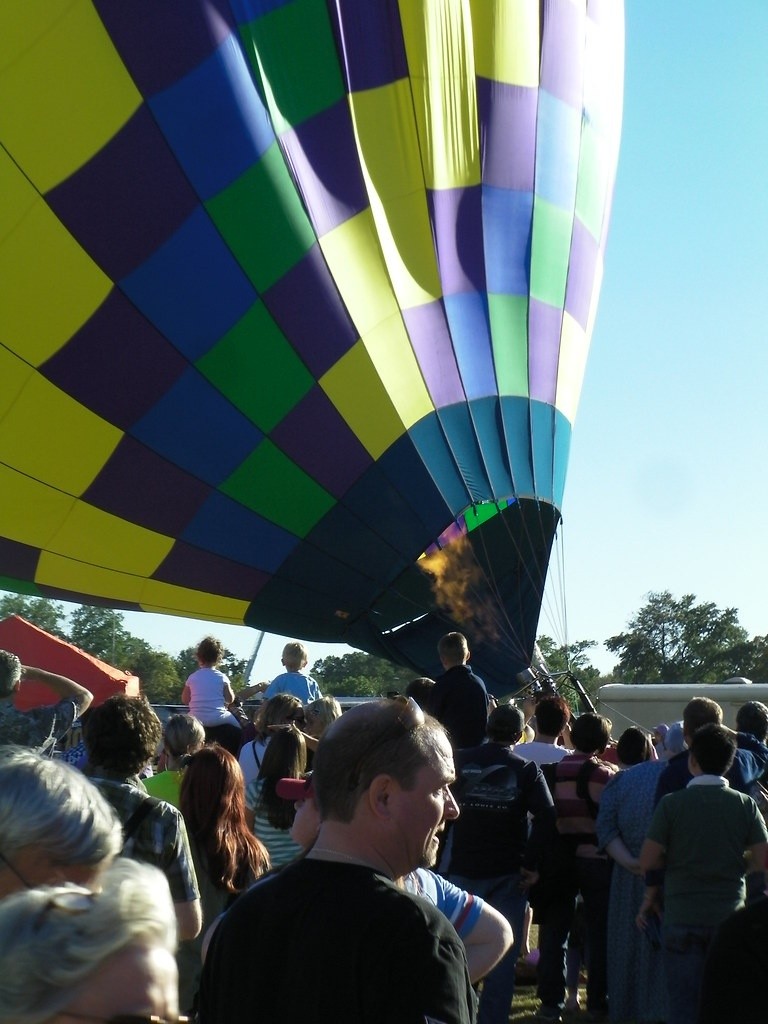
[61,1013,190,1024]
[349,695,424,794]
[34,891,102,936]
[286,714,306,724]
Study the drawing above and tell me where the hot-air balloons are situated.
[0,1,610,745]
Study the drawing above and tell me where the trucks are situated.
[599,676,768,742]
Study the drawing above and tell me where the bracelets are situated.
[644,892,652,900]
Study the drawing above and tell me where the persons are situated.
[201,772,514,984]
[190,696,478,1024]
[181,636,241,728]
[0,742,124,898]
[596,719,687,1024]
[388,630,768,1024]
[141,714,206,812]
[262,643,324,706]
[177,741,272,1009]
[82,695,203,940]
[57,709,149,794]
[0,648,96,753]
[431,703,557,1024]
[205,682,342,783]
[245,721,319,871]
[0,880,192,1024]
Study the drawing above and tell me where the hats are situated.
[653,723,668,736]
[488,704,525,736]
[277,768,316,800]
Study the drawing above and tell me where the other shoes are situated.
[539,1004,561,1021]
[587,996,609,1018]
[565,1000,581,1011]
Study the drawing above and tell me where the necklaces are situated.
[311,846,367,863]
